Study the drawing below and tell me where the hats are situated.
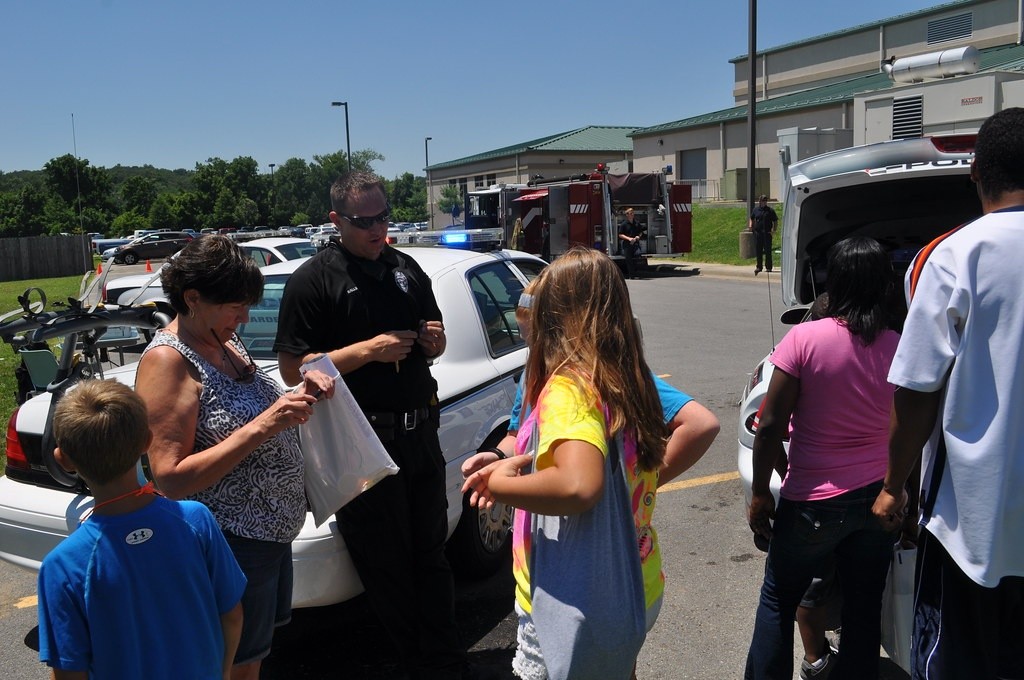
[759,195,769,200]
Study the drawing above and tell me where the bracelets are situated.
[490,448,507,459]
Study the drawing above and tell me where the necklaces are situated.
[81,482,153,522]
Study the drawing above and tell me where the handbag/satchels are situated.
[881,538,918,675]
[529,406,647,680]
[293,370,401,529]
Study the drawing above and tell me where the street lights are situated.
[425,137,432,166]
[269,164,276,207]
[332,101,351,172]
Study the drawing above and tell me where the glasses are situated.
[337,201,393,230]
[211,325,257,381]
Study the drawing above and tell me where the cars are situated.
[737,132,979,529]
[86,220,427,352]
[0,229,644,609]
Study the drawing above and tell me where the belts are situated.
[394,408,429,430]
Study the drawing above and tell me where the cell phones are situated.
[308,388,323,406]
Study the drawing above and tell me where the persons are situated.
[619,208,644,280]
[744,237,902,680]
[748,195,778,272]
[871,105,1024,680]
[133,236,334,680]
[273,174,500,680]
[460,250,719,680]
[38,379,247,679]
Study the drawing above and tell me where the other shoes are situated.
[800,646,836,680]
[623,274,629,279]
[766,268,771,272]
[754,266,762,272]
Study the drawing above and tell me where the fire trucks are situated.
[465,161,694,264]
